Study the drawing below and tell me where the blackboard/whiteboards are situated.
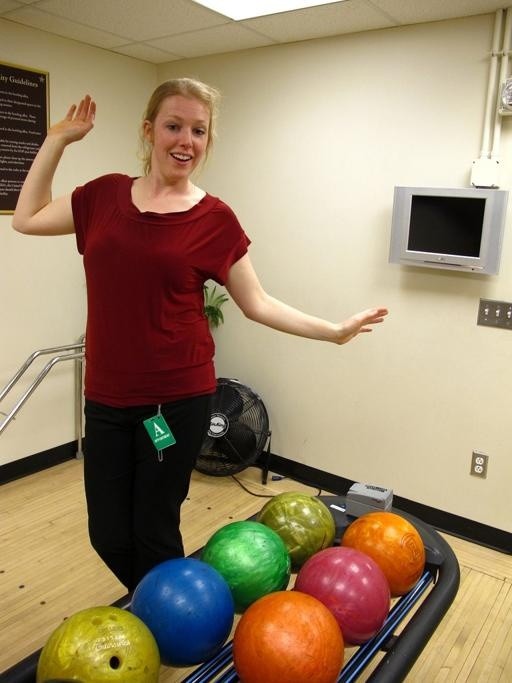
[0,61,51,215]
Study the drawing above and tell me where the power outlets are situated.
[470,451,489,479]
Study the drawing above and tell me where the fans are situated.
[195,376,273,479]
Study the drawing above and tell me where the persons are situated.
[9,75,390,590]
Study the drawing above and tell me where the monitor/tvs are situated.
[388,187,509,276]
